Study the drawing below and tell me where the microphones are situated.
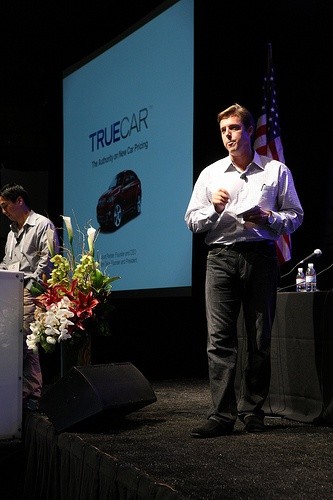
[280,249,322,279]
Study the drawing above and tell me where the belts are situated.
[210,241,275,247]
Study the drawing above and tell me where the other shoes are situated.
[24,396,41,411]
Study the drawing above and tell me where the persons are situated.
[184,102,305,437]
[0,182,60,412]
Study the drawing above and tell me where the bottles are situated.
[296,268,306,292]
[306,263,317,292]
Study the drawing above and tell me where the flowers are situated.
[27,207,123,352]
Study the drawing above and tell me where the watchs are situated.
[266,211,275,224]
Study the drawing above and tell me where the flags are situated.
[253,45,293,266]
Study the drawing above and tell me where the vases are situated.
[74,332,91,368]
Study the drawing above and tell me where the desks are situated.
[234,292,333,426]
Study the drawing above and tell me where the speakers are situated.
[39,361,157,432]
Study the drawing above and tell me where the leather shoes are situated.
[245,417,265,432]
[191,419,234,436]
